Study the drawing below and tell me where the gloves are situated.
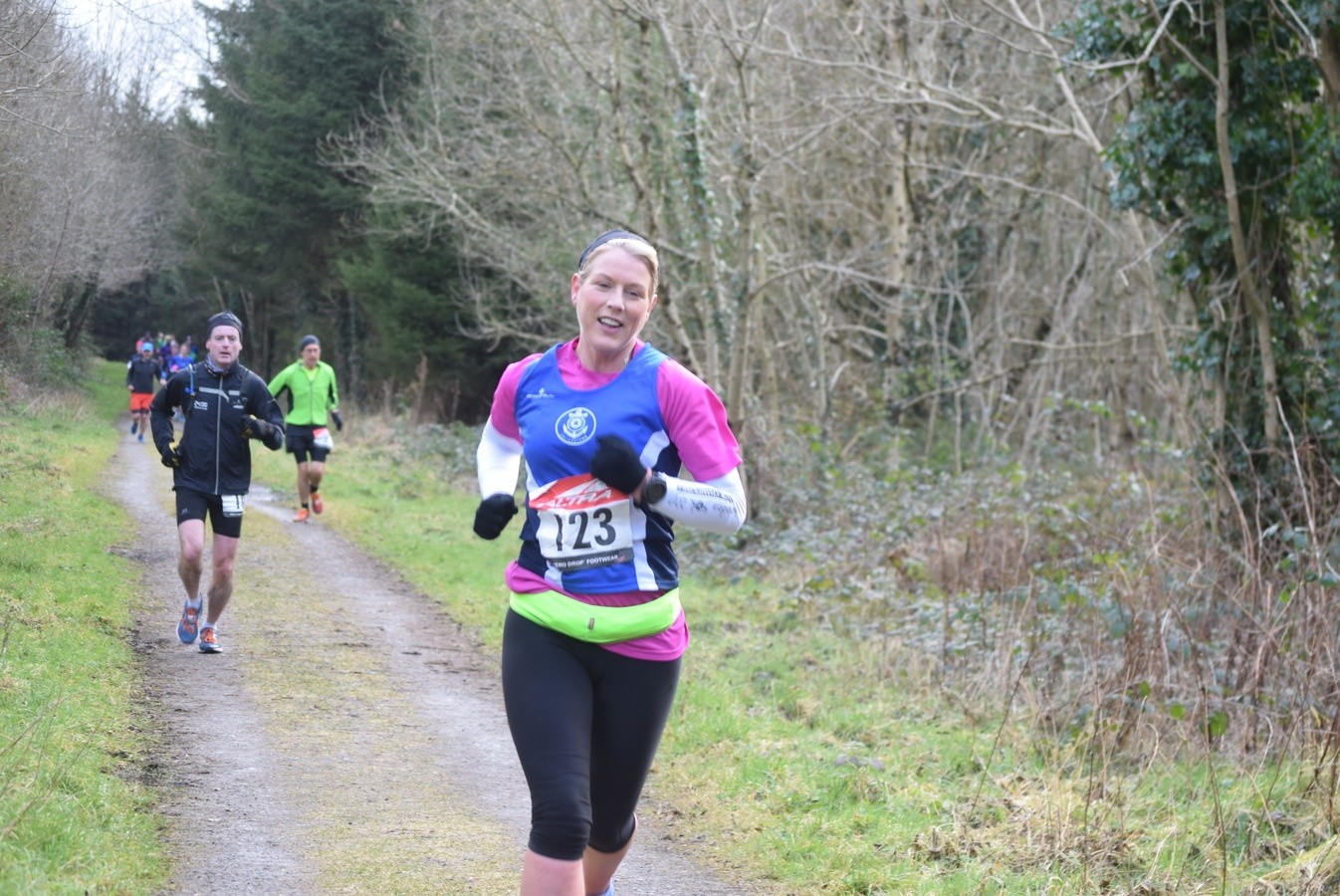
[239,413,269,440]
[591,433,648,495]
[473,494,519,539]
[159,437,180,468]
[331,411,342,430]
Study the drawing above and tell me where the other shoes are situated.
[131,423,138,435]
[310,491,323,513]
[293,508,309,522]
[139,434,143,442]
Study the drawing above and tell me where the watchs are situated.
[632,470,667,509]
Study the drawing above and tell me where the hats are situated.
[142,342,153,351]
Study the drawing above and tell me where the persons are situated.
[126,331,198,443]
[267,334,343,523]
[149,310,286,654]
[475,230,746,896]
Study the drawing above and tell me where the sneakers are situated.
[199,627,222,652]
[178,594,203,644]
